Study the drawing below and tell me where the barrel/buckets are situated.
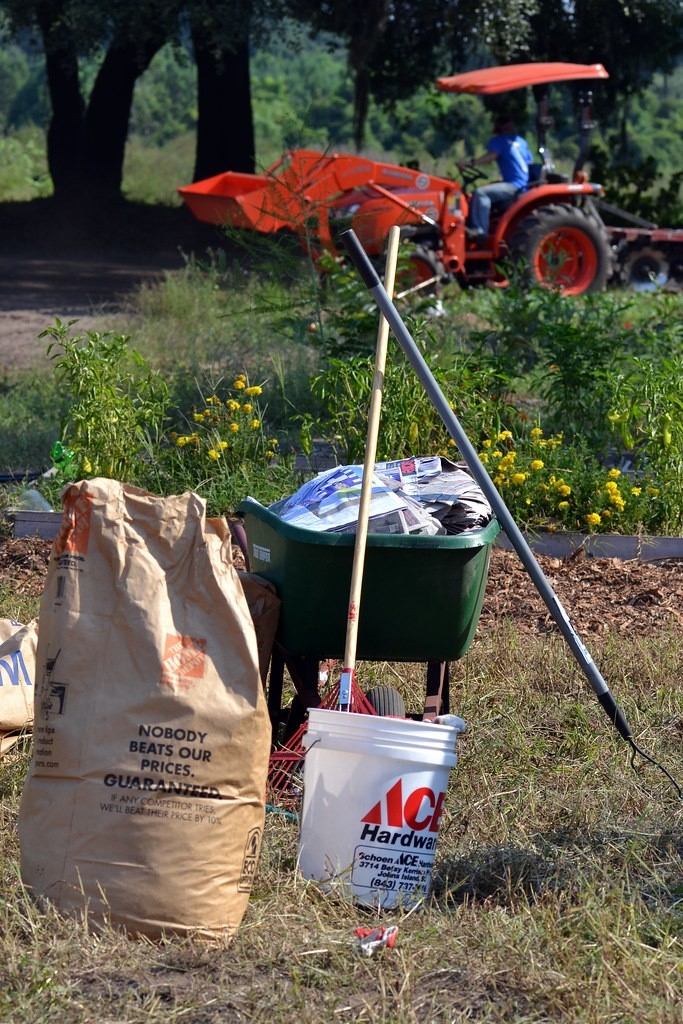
[297,707,459,911]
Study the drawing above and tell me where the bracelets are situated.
[471,159,475,166]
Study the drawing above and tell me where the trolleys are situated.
[226,499,506,725]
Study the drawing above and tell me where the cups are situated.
[19,490,54,512]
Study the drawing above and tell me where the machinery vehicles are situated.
[177,61,682,309]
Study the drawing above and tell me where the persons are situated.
[457,118,534,240]
[332,481,360,501]
